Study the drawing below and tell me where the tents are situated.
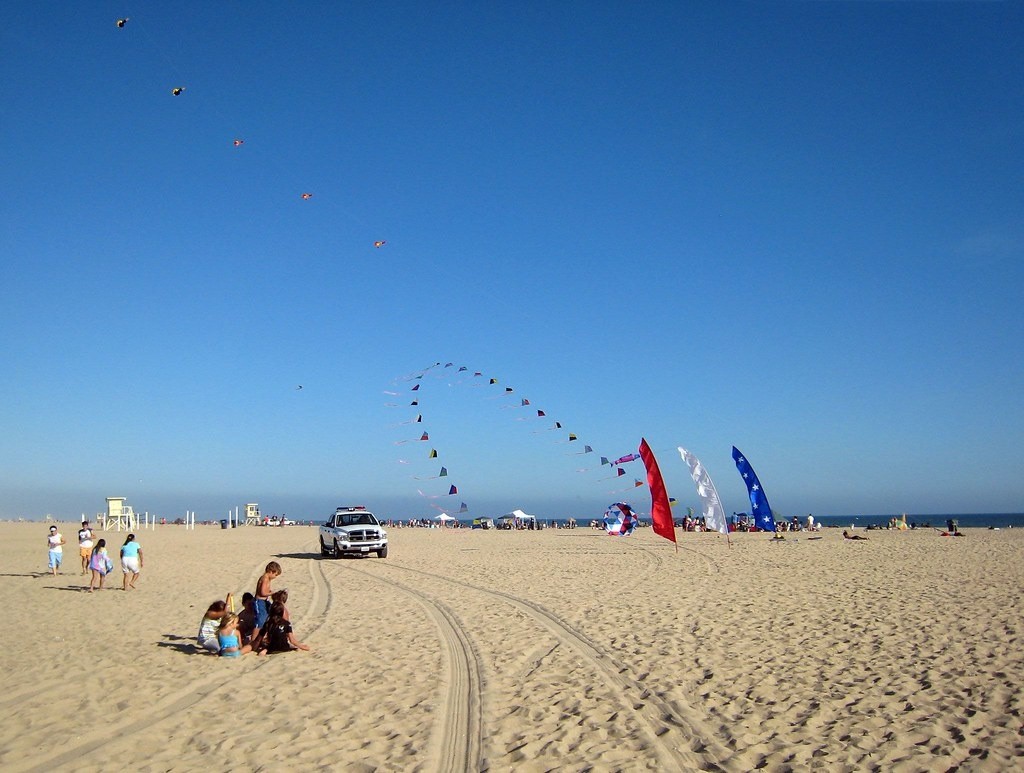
[498,509,536,526]
[435,514,456,525]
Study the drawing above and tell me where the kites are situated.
[375,240,386,250]
[119,18,131,28]
[234,140,245,146]
[379,363,467,513]
[436,362,678,509]
[172,87,184,99]
[301,192,313,201]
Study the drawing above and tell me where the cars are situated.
[266,518,281,526]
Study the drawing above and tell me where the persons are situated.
[940,531,966,537]
[386,518,467,530]
[497,517,576,530]
[47,526,66,576]
[119,534,144,591]
[843,529,868,540]
[867,516,900,531]
[590,519,607,530]
[264,512,313,527]
[79,520,96,576]
[196,561,311,657]
[850,523,854,531]
[89,538,113,593]
[774,514,822,533]
[681,513,755,532]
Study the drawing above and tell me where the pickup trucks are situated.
[279,517,296,526]
[318,506,389,560]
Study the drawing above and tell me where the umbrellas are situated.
[895,520,908,530]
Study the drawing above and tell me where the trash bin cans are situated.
[947,518,958,532]
